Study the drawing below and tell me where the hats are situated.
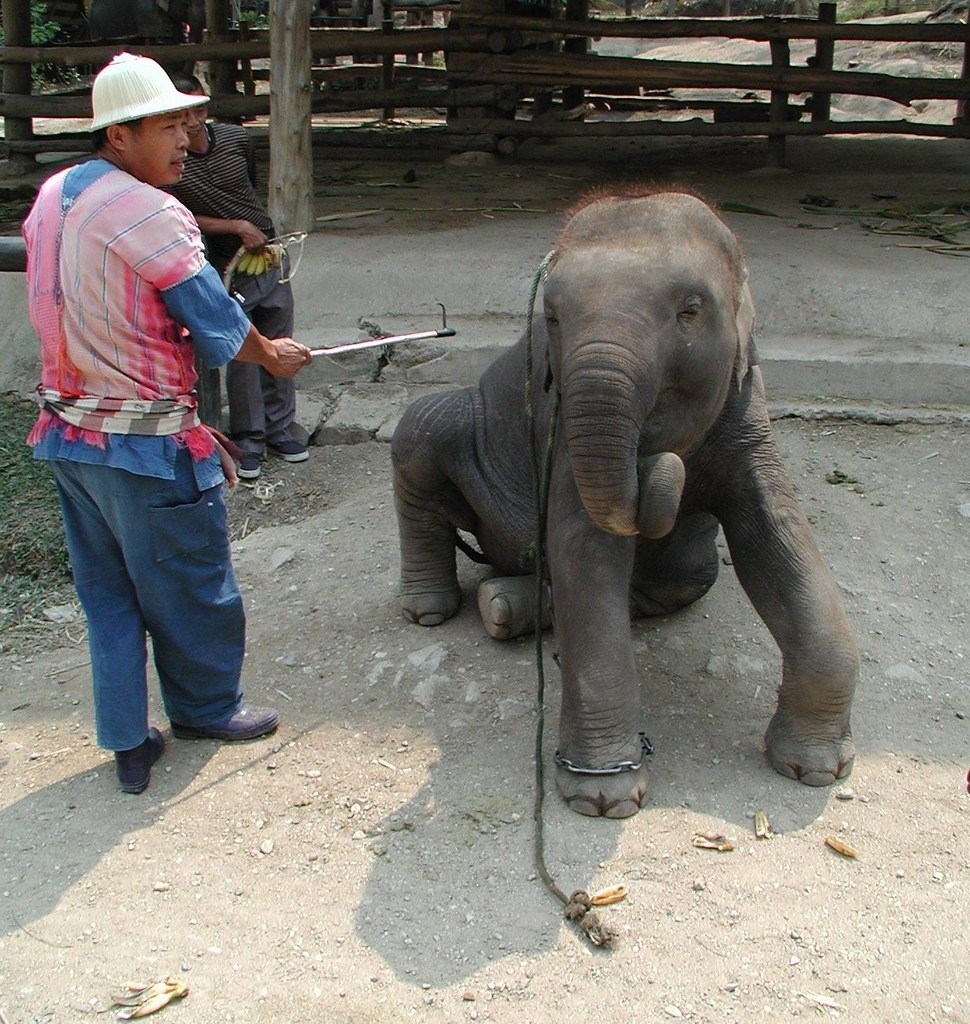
[88,52,214,134]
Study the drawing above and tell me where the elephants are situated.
[390,188,860,818]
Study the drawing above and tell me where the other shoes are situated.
[172,705,282,740]
[266,442,314,463]
[237,456,261,478]
[114,727,164,793]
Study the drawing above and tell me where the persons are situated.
[21,51,312,794]
[170,73,310,478]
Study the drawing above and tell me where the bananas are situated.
[236,243,285,275]
